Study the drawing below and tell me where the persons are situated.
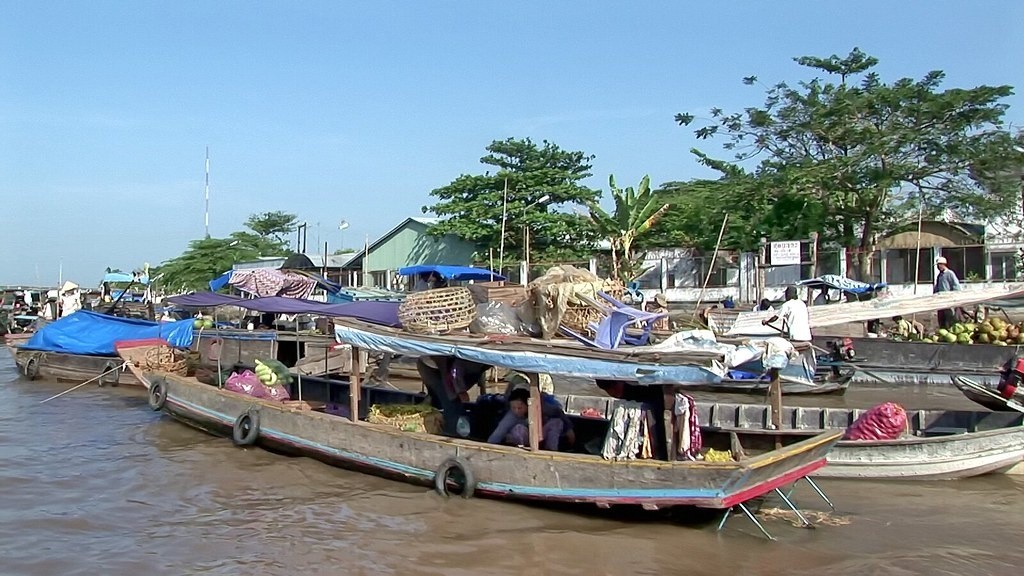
[752,299,774,311]
[762,286,813,342]
[702,305,718,325]
[650,292,669,330]
[887,315,918,337]
[814,285,831,306]
[416,354,493,438]
[484,388,576,451]
[933,256,961,330]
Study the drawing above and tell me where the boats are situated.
[113,289,850,512]
[480,391,1023,478]
[714,331,1024,374]
[1,262,370,387]
[948,350,1024,413]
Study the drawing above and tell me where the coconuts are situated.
[193,319,212,330]
[920,317,1024,347]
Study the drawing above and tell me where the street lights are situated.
[523,195,550,262]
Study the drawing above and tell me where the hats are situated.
[932,257,947,266]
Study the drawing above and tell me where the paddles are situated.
[765,322,889,384]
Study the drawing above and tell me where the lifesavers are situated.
[23,356,39,381]
[98,361,119,388]
[232,410,260,446]
[146,378,167,412]
[433,458,475,500]
